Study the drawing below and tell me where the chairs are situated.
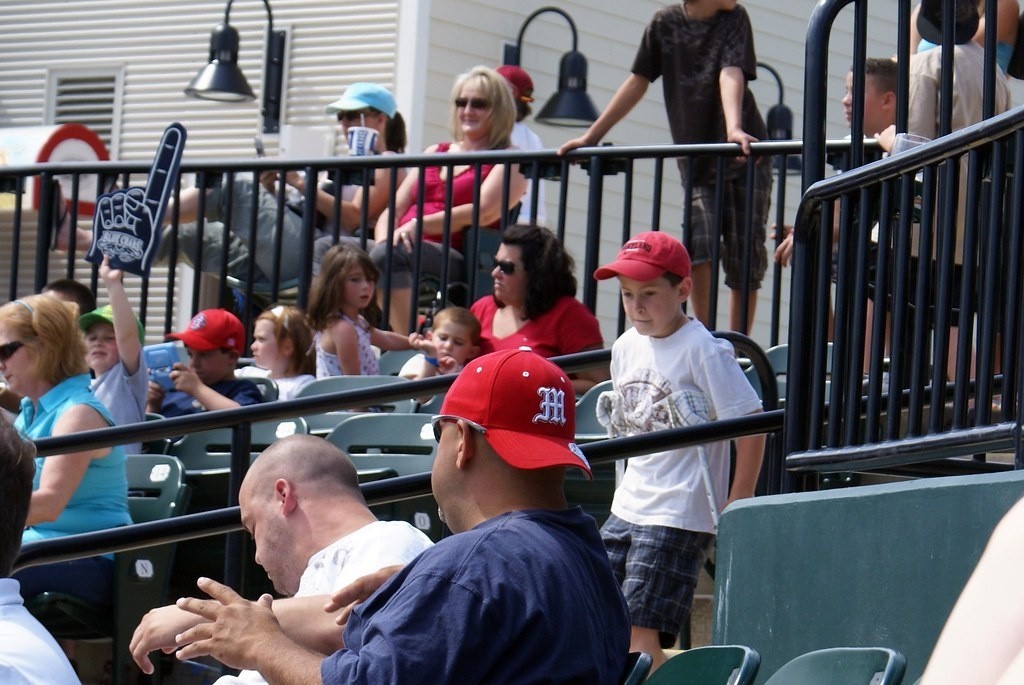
[20,197,907,685]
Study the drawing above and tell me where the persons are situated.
[593,231,766,681]
[130,346,634,685]
[0,414,83,685]
[556,0,774,342]
[305,65,528,342]
[51,81,407,308]
[775,0,1024,407]
[0,225,607,639]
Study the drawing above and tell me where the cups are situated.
[347,126,379,163]
[890,134,934,222]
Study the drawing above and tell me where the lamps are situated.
[183,0,288,133]
[743,62,801,170]
[501,4,602,129]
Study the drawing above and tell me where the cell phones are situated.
[254,137,266,160]
[147,372,177,389]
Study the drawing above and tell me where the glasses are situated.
[432,415,487,443]
[338,109,370,120]
[489,258,527,275]
[454,98,493,112]
[0,333,40,363]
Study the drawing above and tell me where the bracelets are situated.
[412,216,417,226]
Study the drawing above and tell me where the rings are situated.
[401,233,409,240]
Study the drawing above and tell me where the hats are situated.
[916,0,980,44]
[442,345,591,479]
[164,310,244,356]
[77,304,146,349]
[594,233,693,280]
[323,82,397,119]
[494,66,536,101]
